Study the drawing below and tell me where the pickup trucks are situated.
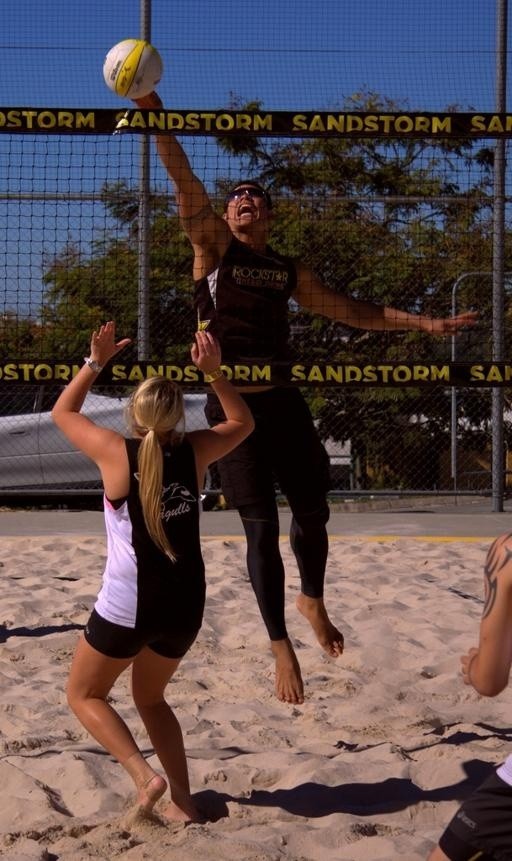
[1,382,353,509]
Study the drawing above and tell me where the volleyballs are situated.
[103,39,163,99]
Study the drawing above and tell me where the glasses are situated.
[227,188,267,198]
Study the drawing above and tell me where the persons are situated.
[49,321,258,828]
[425,529,512,861]
[130,90,484,706]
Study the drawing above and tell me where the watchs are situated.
[83,357,103,375]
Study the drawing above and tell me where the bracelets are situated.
[203,367,223,386]
